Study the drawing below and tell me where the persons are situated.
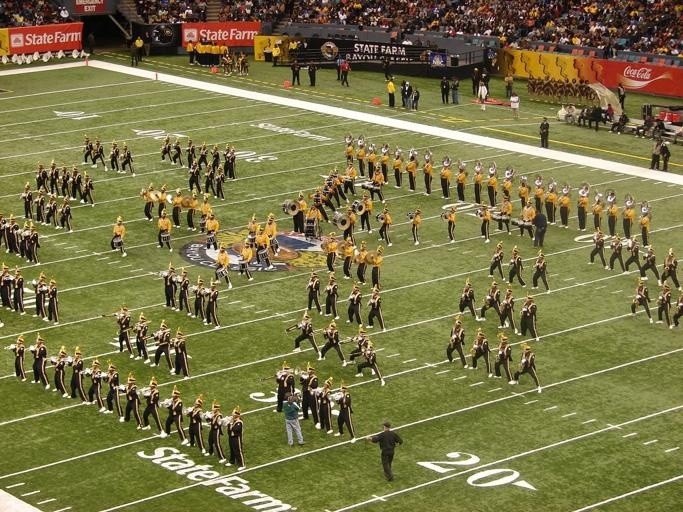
[364,421,404,481]
[0,128,683,474]
[378,0,683,172]
[0,1,379,90]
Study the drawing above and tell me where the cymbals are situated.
[139,187,193,209]
[320,240,376,266]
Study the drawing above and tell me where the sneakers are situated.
[463,364,477,369]
[346,319,352,323]
[650,318,677,329]
[318,311,339,319]
[5,308,26,316]
[359,323,373,328]
[318,351,325,361]
[497,322,539,341]
[163,303,220,330]
[33,313,60,325]
[157,432,245,470]
[355,373,363,377]
[488,373,502,379]
[371,369,376,375]
[476,316,486,322]
[22,378,95,405]
[293,347,300,352]
[380,379,385,386]
[99,407,151,430]
[508,379,520,385]
[537,386,542,393]
[350,438,356,443]
[342,359,356,367]
[169,368,178,375]
[130,354,159,367]
[298,416,305,420]
[314,422,344,437]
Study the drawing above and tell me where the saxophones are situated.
[591,227,682,314]
[449,254,541,373]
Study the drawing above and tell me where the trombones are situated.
[295,319,384,357]
[259,367,293,382]
[101,311,185,348]
[305,271,378,309]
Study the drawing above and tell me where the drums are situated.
[161,233,172,243]
[239,233,276,270]
[407,212,415,221]
[283,178,386,239]
[114,236,123,248]
[440,211,450,222]
[207,232,215,244]
[215,268,227,278]
[199,218,207,228]
[491,214,533,229]
[476,210,485,219]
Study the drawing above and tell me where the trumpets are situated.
[156,270,213,295]
[2,346,235,426]
[295,367,346,402]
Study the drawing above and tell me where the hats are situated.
[60,345,136,382]
[0,213,37,230]
[311,272,379,294]
[139,315,183,337]
[169,267,216,287]
[16,337,44,344]
[150,377,240,417]
[1,265,22,274]
[282,361,348,392]
[456,321,462,326]
[477,331,530,351]
[39,274,56,285]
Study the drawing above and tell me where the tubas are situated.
[344,133,417,167]
[471,157,557,200]
[560,183,653,223]
[422,147,466,180]
